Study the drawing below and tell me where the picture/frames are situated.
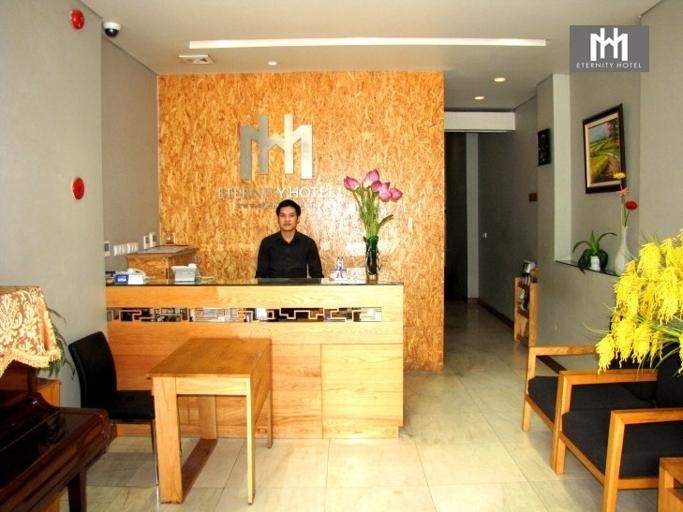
[581,103,624,197]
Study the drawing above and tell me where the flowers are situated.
[613,173,637,227]
[596,232,682,375]
[343,169,401,239]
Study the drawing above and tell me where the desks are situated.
[143,335,273,504]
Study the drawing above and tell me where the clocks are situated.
[535,130,551,166]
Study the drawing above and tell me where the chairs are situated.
[520,315,679,470]
[67,331,159,489]
[556,351,682,512]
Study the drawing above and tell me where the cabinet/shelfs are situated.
[512,262,537,350]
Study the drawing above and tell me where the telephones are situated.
[112,267,145,285]
[170,263,201,282]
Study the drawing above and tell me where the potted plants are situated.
[572,229,615,275]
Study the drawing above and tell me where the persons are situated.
[255,199,324,278]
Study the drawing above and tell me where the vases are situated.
[614,224,637,277]
[364,236,379,281]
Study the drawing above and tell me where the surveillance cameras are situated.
[102,21,121,37]
